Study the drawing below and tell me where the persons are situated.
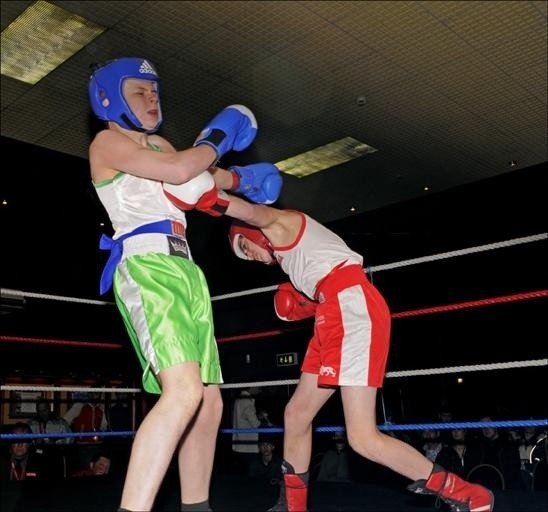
[162,170,495,512]
[0,392,111,481]
[316,432,351,483]
[230,388,261,478]
[88,56,283,512]
[400,405,548,492]
[239,432,283,481]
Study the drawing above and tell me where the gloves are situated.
[161,169,230,219]
[226,162,284,207]
[272,280,334,323]
[193,103,260,159]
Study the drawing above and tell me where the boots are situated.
[408,459,495,511]
[267,458,311,511]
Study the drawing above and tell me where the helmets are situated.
[229,214,280,266]
[88,57,165,136]
